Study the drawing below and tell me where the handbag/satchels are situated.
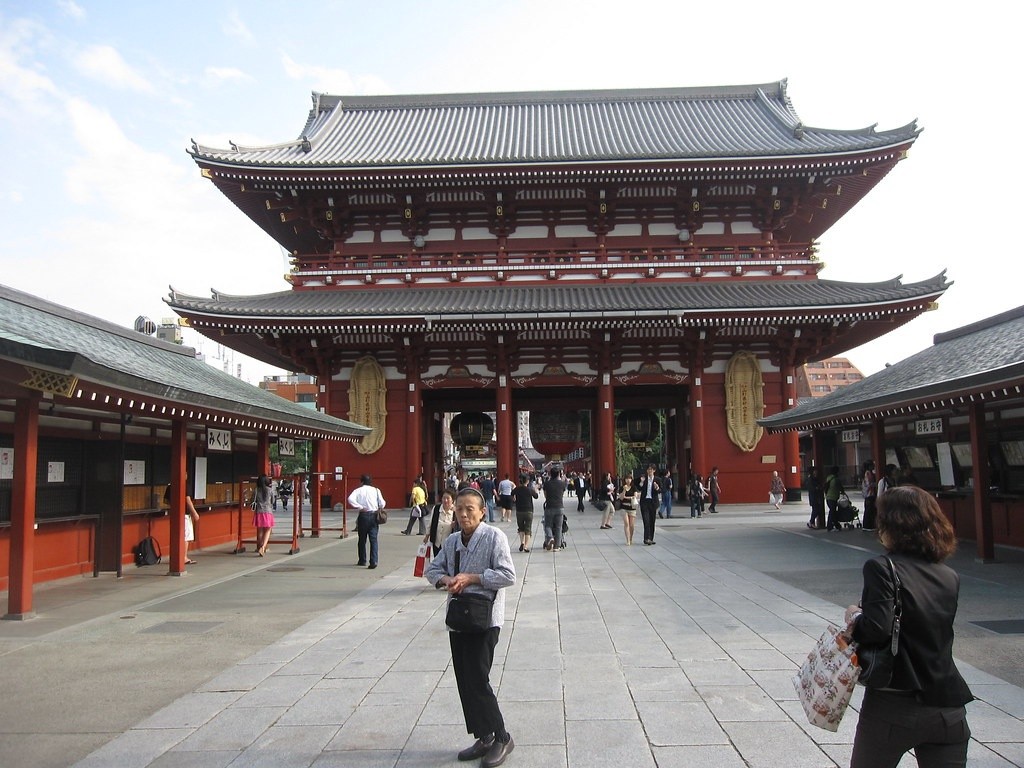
[631,496,639,509]
[595,499,606,510]
[414,543,431,579]
[445,592,494,632]
[824,481,831,494]
[250,502,257,512]
[613,498,622,510]
[411,505,421,517]
[791,612,863,733]
[374,507,387,524]
[769,492,776,505]
[855,555,903,688]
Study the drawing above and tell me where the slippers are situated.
[186,559,197,564]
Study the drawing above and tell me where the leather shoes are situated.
[481,732,514,768]
[457,736,497,761]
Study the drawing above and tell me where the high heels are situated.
[259,546,264,556]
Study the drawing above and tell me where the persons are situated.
[861,459,919,531]
[511,473,538,552]
[251,474,275,558]
[574,472,587,512]
[348,473,387,569]
[770,471,786,509]
[424,489,462,558]
[526,471,593,497]
[689,466,722,519]
[636,464,663,546]
[163,472,199,564]
[618,474,639,546]
[600,472,617,529]
[808,464,856,532]
[425,487,516,768]
[401,473,429,535]
[658,468,673,519]
[448,472,516,523]
[272,478,310,512]
[544,467,566,552]
[844,484,975,768]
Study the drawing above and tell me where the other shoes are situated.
[644,539,651,545]
[658,508,718,519]
[401,530,410,535]
[416,532,423,535]
[652,540,656,544]
[484,509,612,553]
[806,520,879,534]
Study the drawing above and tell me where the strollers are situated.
[541,500,568,549]
[835,493,862,530]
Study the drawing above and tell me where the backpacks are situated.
[136,536,162,566]
